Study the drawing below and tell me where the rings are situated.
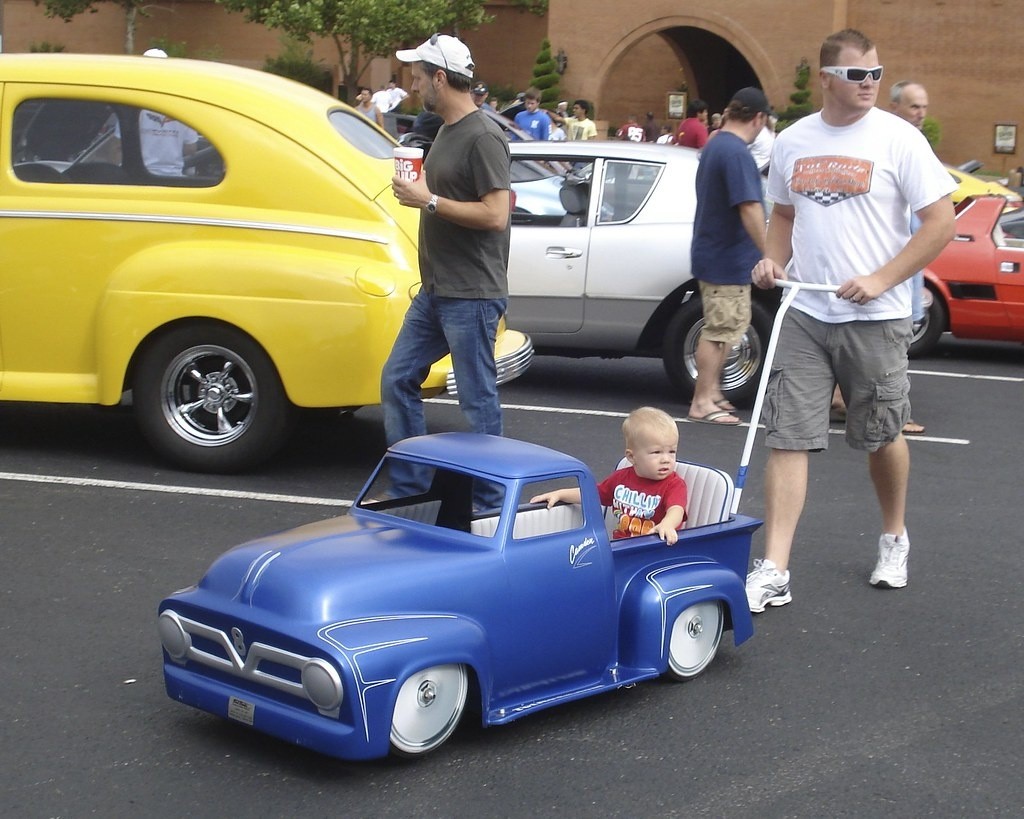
[850,298,855,303]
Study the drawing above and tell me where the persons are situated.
[530,407,688,546]
[675,99,721,149]
[470,81,497,113]
[370,80,409,114]
[703,106,778,174]
[548,101,568,140]
[113,48,198,178]
[616,112,679,146]
[369,32,511,514]
[829,80,928,434]
[544,99,597,140]
[687,87,768,426]
[512,86,550,141]
[745,26,956,612]
[355,87,384,130]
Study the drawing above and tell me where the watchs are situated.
[425,195,438,215]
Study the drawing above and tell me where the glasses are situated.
[820,65,884,84]
[430,32,475,70]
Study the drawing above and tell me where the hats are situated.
[396,33,475,79]
[733,87,780,119]
[473,81,488,94]
[143,48,167,58]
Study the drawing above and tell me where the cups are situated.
[393,146,424,183]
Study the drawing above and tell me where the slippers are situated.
[687,397,742,425]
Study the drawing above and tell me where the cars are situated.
[941,161,1023,214]
[397,132,779,404]
[0,52,535,475]
[157,430,765,761]
[907,193,1024,359]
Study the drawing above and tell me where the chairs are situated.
[559,182,590,227]
[601,455,734,540]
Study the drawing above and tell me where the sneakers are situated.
[744,559,792,613]
[869,525,910,589]
